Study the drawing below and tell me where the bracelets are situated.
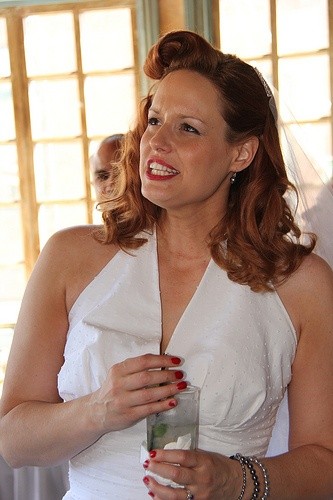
[230,455,260,500]
[233,453,247,500]
[248,455,270,500]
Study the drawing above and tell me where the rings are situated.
[186,487,194,499]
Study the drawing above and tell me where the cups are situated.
[146,384,200,488]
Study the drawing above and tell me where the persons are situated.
[95,135,125,200]
[0,31,333,499]
